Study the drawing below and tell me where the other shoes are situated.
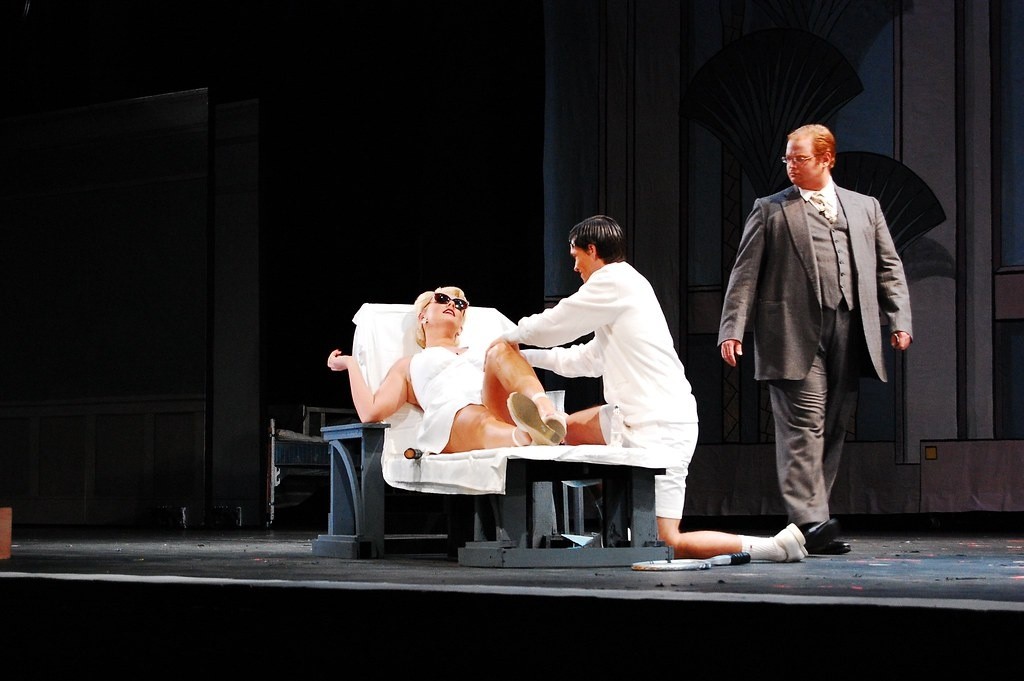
[775,523,808,563]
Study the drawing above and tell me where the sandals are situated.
[531,391,566,437]
[506,391,562,447]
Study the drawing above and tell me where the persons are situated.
[327,286,566,454]
[717,124,913,555]
[482,215,808,563]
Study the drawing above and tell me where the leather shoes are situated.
[817,538,852,555]
[802,517,841,551]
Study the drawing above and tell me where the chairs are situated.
[312,302,676,568]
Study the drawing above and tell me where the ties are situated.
[809,191,838,225]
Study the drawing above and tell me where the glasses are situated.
[423,292,468,311]
[781,153,823,165]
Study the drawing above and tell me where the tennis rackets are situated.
[631,548,753,572]
